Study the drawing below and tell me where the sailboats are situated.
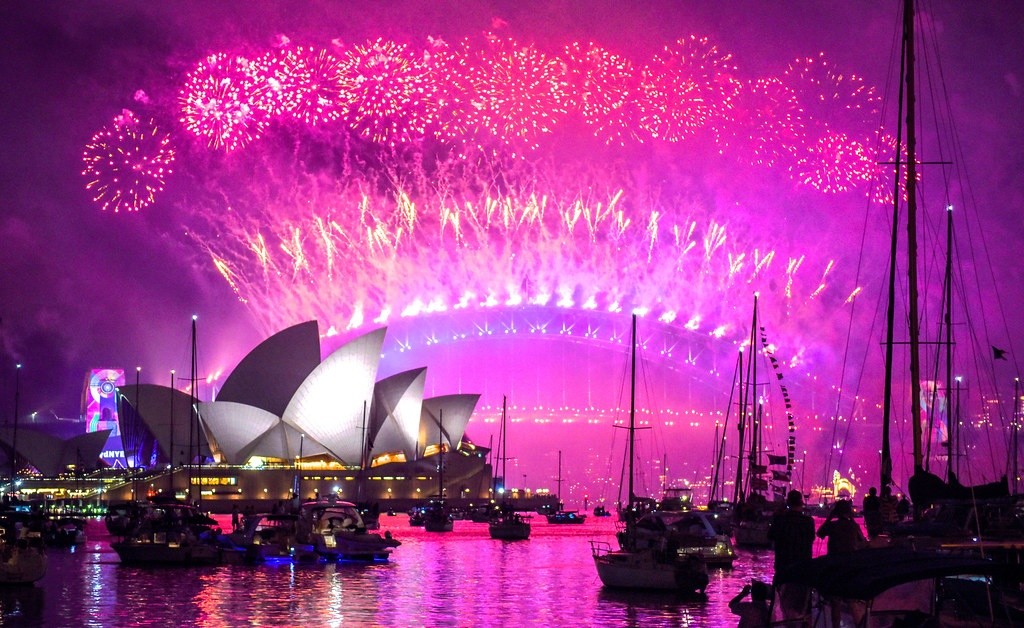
[589,0,1023,627]
[489,396,530,542]
[546,450,587,524]
[472,435,500,523]
[424,409,454,532]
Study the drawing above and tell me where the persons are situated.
[767,488,815,628]
[862,486,910,538]
[817,499,865,628]
[232,492,339,533]
[728,581,777,628]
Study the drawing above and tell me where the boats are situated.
[0,491,401,586]
[410,511,425,526]
